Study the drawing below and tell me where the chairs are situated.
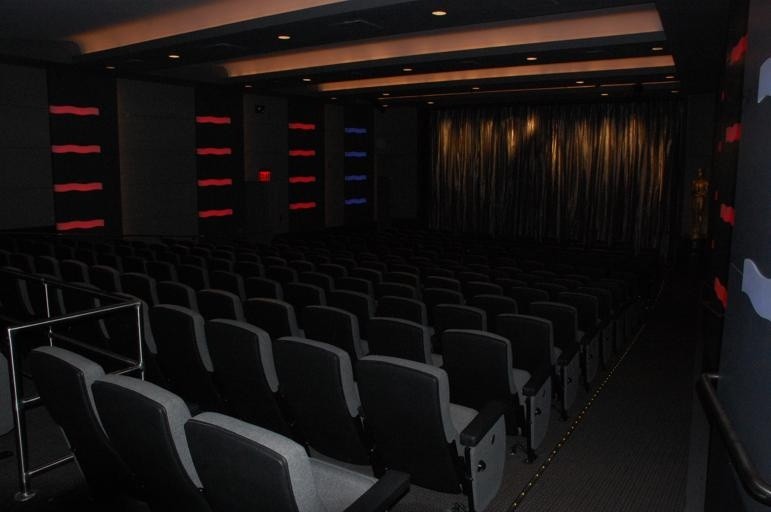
[353,354,507,512]
[273,336,363,446]
[494,313,581,423]
[208,315,292,431]
[365,318,432,365]
[147,303,213,380]
[441,328,552,464]
[0,227,632,328]
[26,344,124,474]
[183,410,411,512]
[533,300,603,379]
[89,373,208,511]
